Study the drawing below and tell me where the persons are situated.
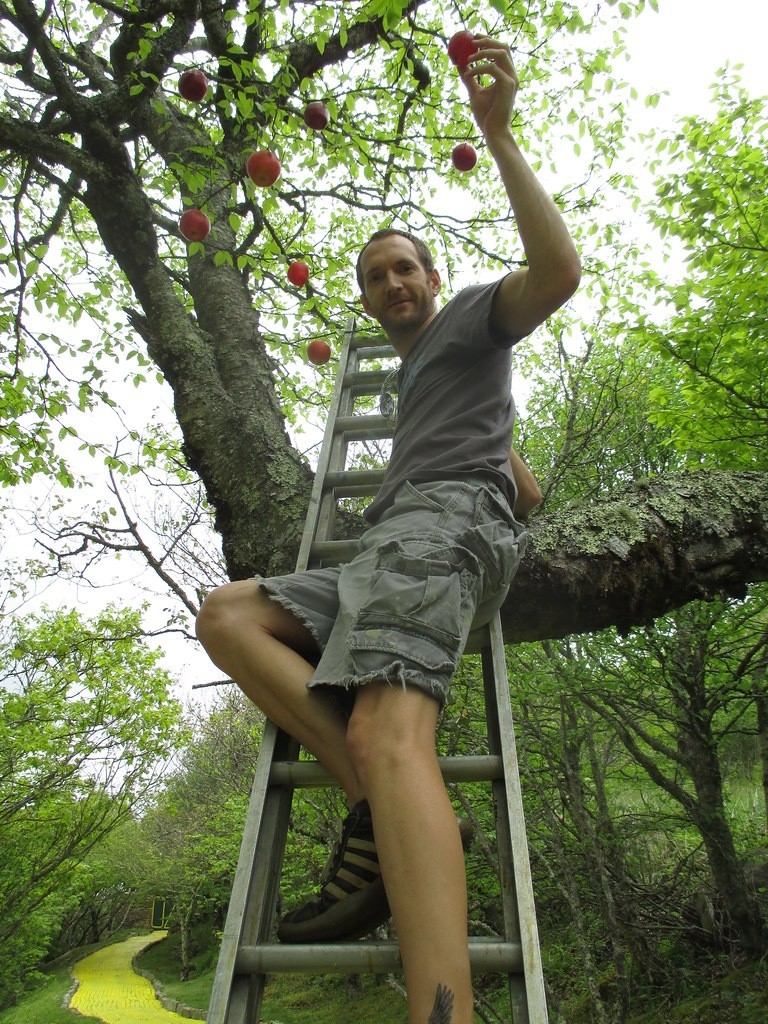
[195,34,581,1024]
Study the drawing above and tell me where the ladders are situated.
[204,319,550,1024]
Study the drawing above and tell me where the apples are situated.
[452,143,477,171]
[179,208,210,242]
[446,29,479,66]
[307,341,331,365]
[178,69,207,101]
[247,151,281,187]
[288,262,309,286]
[304,102,330,130]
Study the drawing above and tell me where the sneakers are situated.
[277,797,473,945]
